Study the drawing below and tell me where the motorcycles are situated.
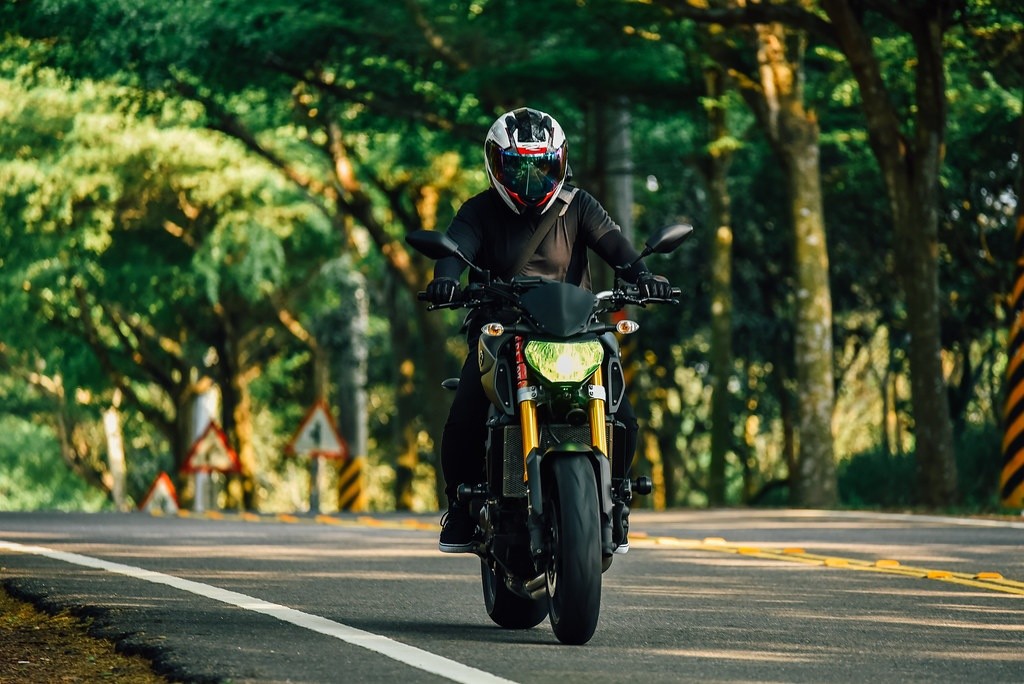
[403,222,694,644]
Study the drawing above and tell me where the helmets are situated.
[482,107,570,220]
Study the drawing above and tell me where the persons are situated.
[425,107,673,555]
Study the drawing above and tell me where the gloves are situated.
[637,273,671,299]
[424,278,463,310]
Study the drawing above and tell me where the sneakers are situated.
[438,509,477,552]
[613,538,629,555]
